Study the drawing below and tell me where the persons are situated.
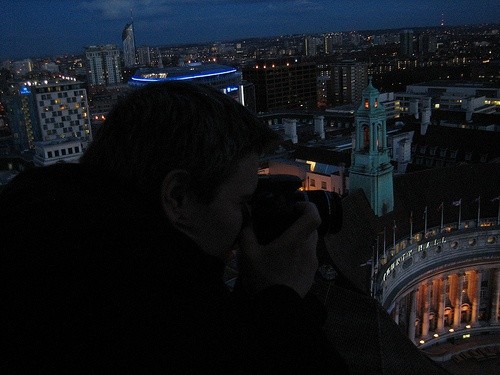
[0,79,324,375]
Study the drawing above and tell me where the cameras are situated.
[244,173,343,247]
